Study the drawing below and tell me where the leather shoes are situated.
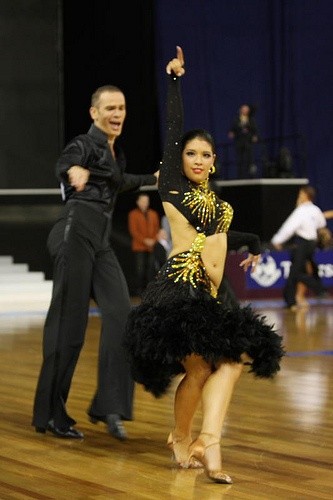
[46,415,84,439]
[105,410,128,440]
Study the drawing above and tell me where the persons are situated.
[227,101,259,179]
[267,187,333,312]
[32,88,160,441]
[120,45,288,483]
[126,194,174,298]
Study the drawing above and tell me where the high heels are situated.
[167,430,235,483]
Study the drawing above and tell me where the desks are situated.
[225,250,333,299]
